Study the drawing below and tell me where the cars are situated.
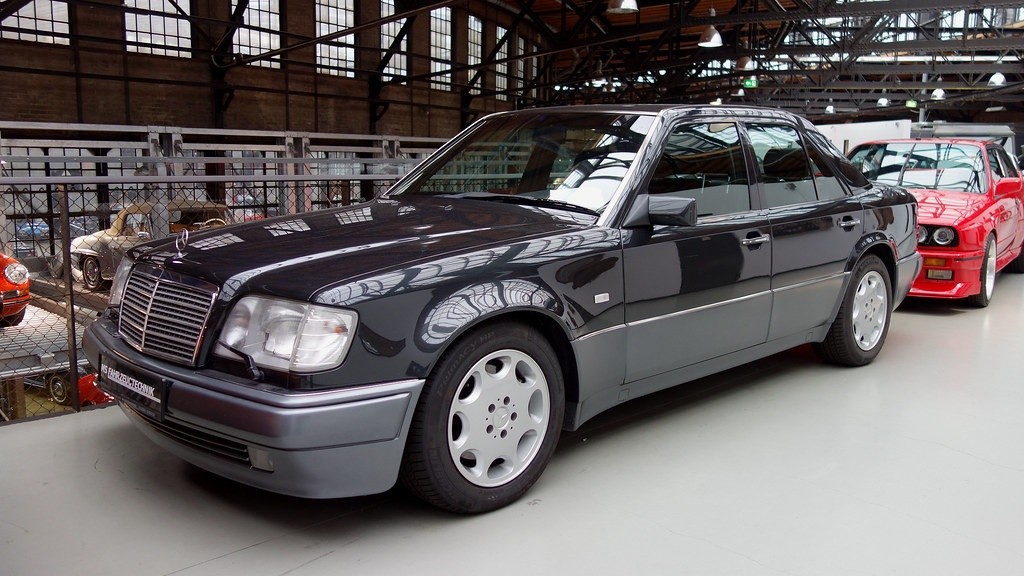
[843,137,1024,310]
[66,196,242,290]
[80,101,925,513]
[16,218,51,240]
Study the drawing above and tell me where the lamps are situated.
[824,98,836,114]
[929,76,946,100]
[733,56,753,70]
[620,0,639,12]
[875,88,890,107]
[697,5,723,48]
[987,60,1007,87]
